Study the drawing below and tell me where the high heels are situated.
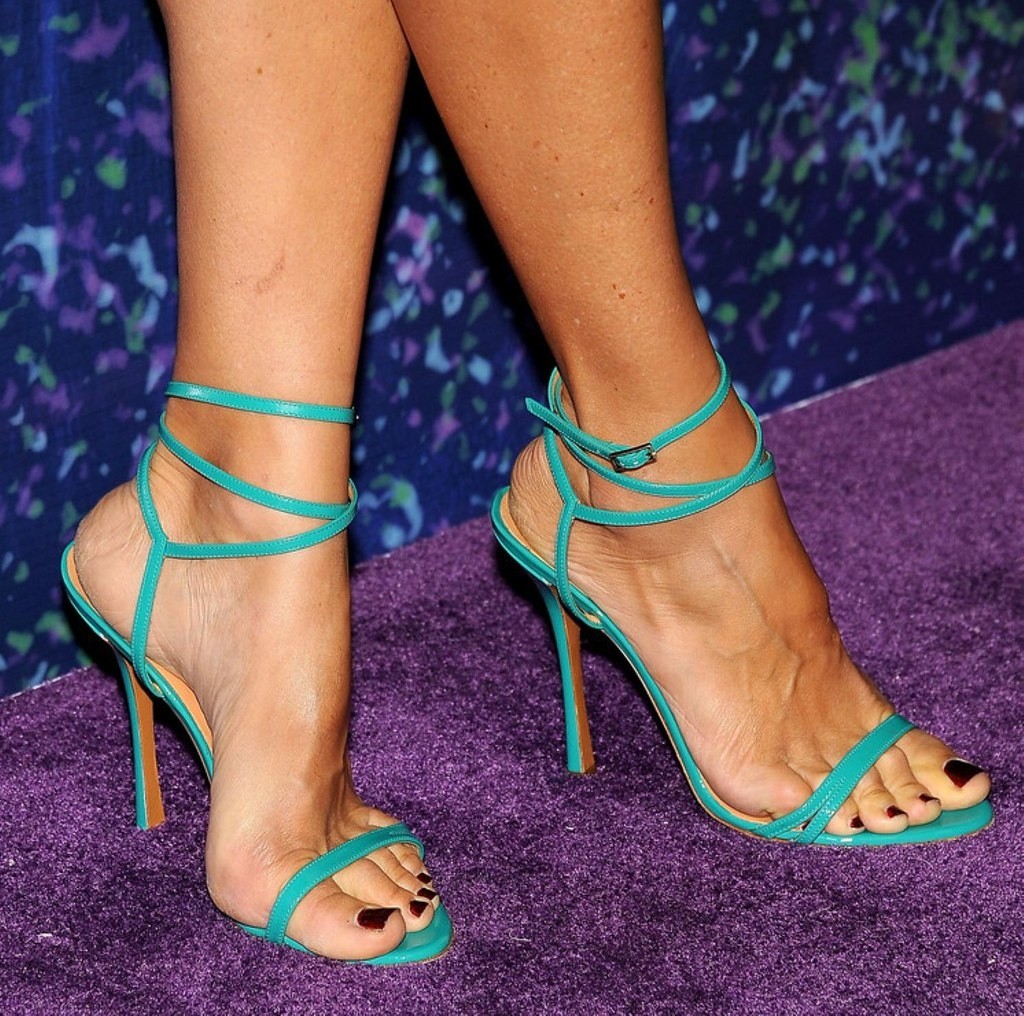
[490,349,991,848]
[61,382,450,964]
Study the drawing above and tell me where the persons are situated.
[61,0,989,965]
